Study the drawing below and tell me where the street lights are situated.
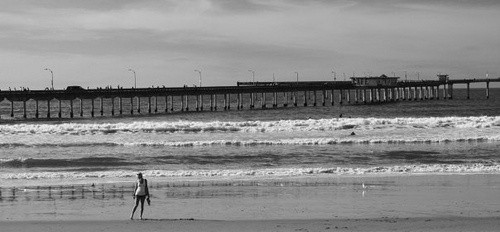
[194,68,202,88]
[294,71,298,85]
[247,69,255,82]
[44,68,55,91]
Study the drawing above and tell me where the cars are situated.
[67,85,87,90]
[129,69,137,88]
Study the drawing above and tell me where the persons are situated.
[87,86,89,90]
[152,85,154,88]
[162,85,165,88]
[184,84,188,87]
[132,86,134,88]
[97,86,112,89]
[157,85,159,88]
[117,84,122,89]
[130,172,149,220]
[45,87,49,91]
[9,86,29,92]
[195,85,197,87]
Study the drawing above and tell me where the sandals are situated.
[146,198,151,205]
